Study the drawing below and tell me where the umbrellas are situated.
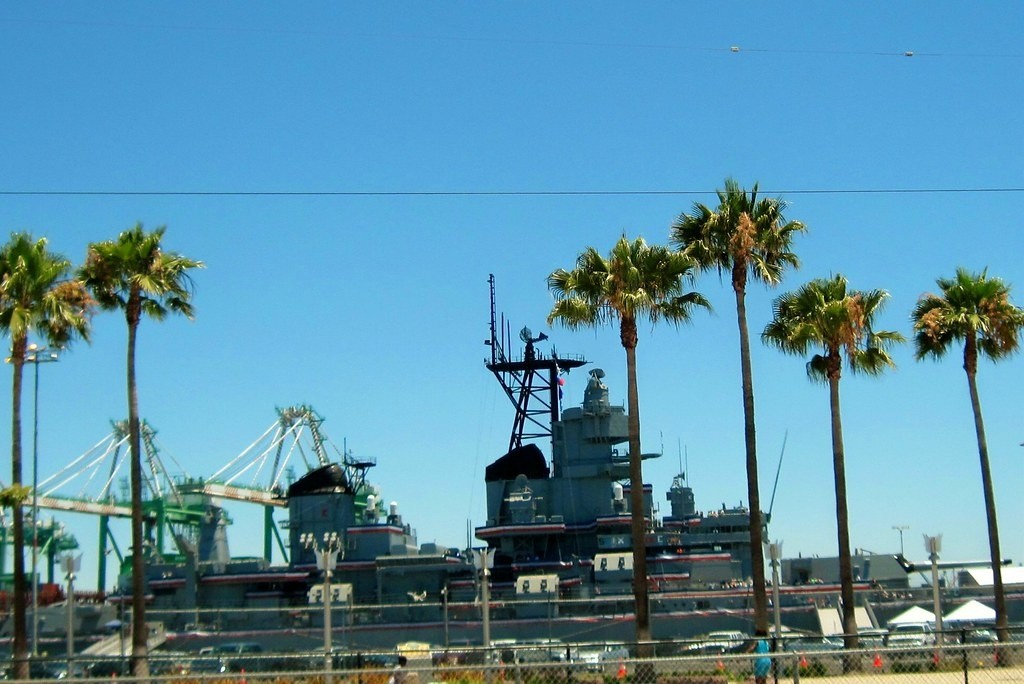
[769,624,791,632]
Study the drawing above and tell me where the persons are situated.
[720,576,753,590]
[708,510,724,517]
[744,630,771,684]
[393,656,407,684]
[856,574,912,600]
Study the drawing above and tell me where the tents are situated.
[886,599,996,623]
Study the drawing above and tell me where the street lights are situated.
[24,344,60,651]
[891,525,909,558]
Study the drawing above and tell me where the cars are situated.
[32,651,130,678]
[858,623,936,653]
[703,631,747,654]
[784,634,847,652]
[147,644,353,674]
[433,639,626,670]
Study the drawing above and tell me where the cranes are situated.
[481,272,594,473]
[192,404,330,509]
[22,421,235,527]
